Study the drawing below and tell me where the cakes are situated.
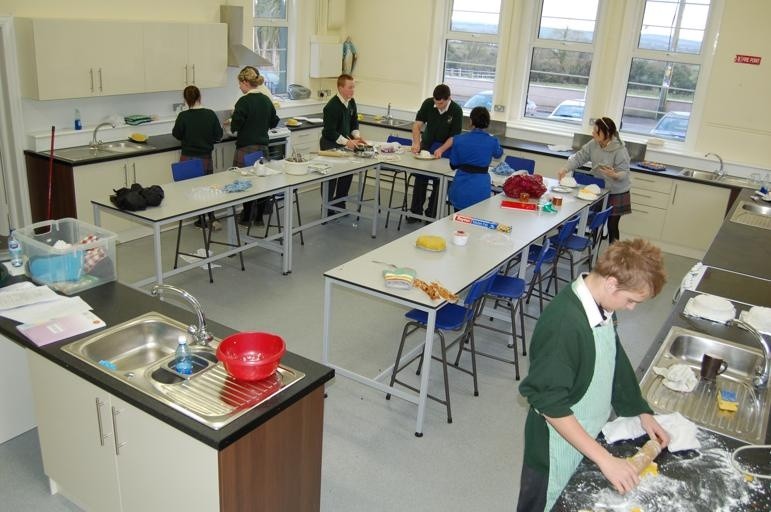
[561,176,601,200]
[420,149,431,158]
[416,236,446,250]
[288,118,297,125]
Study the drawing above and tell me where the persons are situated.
[516,240,671,512]
[558,117,632,244]
[224,66,279,226]
[232,66,274,214]
[448,107,503,213]
[320,75,367,225]
[408,85,462,223]
[172,86,223,230]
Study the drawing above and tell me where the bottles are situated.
[452,230,470,246]
[8,226,23,267]
[259,156,265,165]
[176,335,194,374]
[74,109,84,130]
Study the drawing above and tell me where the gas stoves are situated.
[268,128,291,140]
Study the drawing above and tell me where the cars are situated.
[258,71,279,89]
[459,89,538,118]
[643,110,691,142]
[545,97,584,124]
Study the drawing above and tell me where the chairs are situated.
[90,136,537,302]
[325,171,615,438]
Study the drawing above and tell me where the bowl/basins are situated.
[284,157,310,176]
[215,330,287,383]
[355,145,374,158]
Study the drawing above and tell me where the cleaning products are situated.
[74,108,83,130]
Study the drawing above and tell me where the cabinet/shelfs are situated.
[12,16,143,100]
[34,354,324,512]
[356,123,415,196]
[143,21,227,93]
[22,148,177,242]
[618,169,675,249]
[296,131,323,192]
[659,180,733,260]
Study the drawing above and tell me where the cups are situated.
[701,351,729,383]
[521,193,531,202]
[421,150,431,157]
[537,200,545,210]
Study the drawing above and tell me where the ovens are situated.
[269,138,289,161]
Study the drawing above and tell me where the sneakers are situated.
[195,222,207,228]
[237,214,252,226]
[254,219,264,226]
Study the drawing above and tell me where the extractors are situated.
[220,6,277,69]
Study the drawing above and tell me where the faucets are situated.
[151,284,213,347]
[725,318,771,388]
[91,122,116,145]
[705,152,725,176]
[384,102,394,120]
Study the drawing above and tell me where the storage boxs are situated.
[11,216,121,295]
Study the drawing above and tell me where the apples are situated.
[504,174,547,198]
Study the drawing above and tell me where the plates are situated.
[284,123,303,127]
[552,176,602,201]
[553,197,564,206]
[416,156,438,161]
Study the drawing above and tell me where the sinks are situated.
[658,325,771,381]
[375,118,414,127]
[678,167,720,181]
[739,200,771,219]
[59,310,198,375]
[144,348,221,386]
[97,140,157,154]
